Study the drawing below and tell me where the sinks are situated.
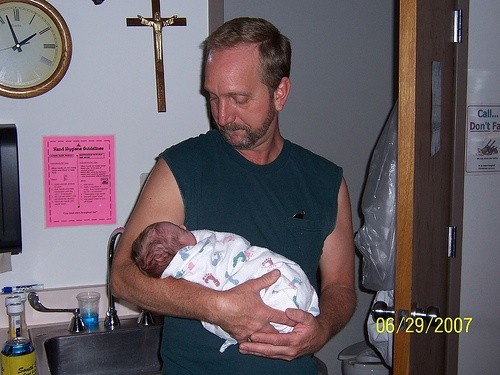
[35,322,163,375]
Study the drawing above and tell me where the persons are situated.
[109,17,357,375]
[137,12,177,60]
[130,221,320,353]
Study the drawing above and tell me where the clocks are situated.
[0,0,72,99]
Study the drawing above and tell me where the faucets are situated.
[104,228,124,329]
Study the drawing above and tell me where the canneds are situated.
[2,337,35,357]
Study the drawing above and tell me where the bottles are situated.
[0,290,38,375]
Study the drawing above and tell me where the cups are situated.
[76,291,100,328]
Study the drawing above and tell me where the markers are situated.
[1,283,45,294]
